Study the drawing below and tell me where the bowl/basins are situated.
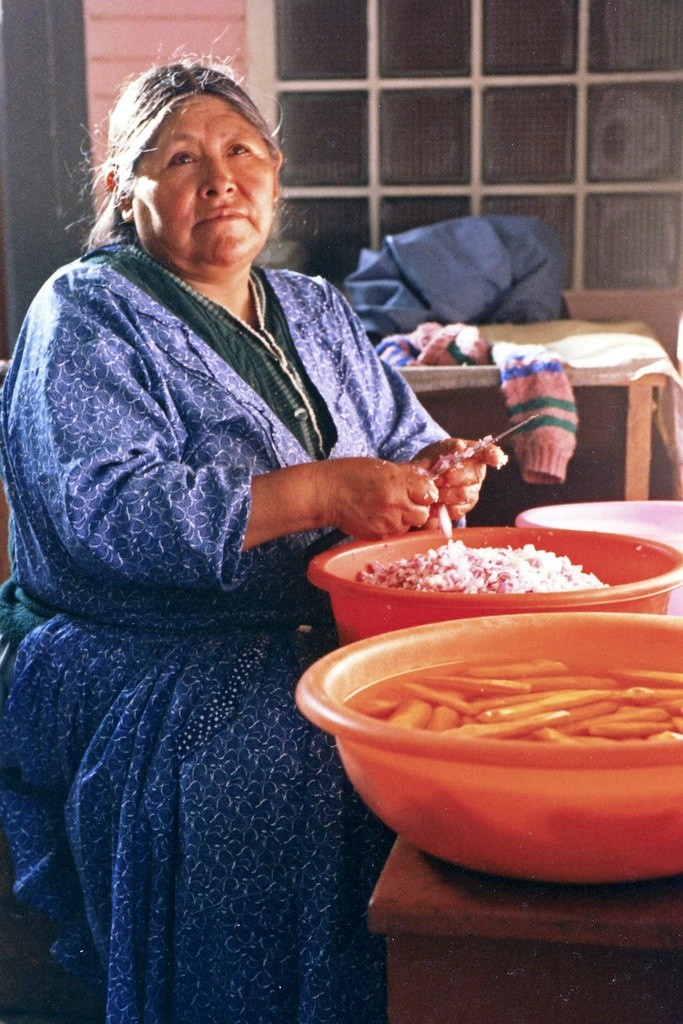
[294,612,683,884]
[308,528,683,649]
[515,501,683,616]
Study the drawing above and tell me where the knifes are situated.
[309,415,537,556]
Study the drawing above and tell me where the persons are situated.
[0,62,504,1023]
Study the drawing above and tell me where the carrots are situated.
[359,658,681,747]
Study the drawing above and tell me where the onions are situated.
[349,438,612,592]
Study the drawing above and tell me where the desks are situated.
[364,834,683,1024]
